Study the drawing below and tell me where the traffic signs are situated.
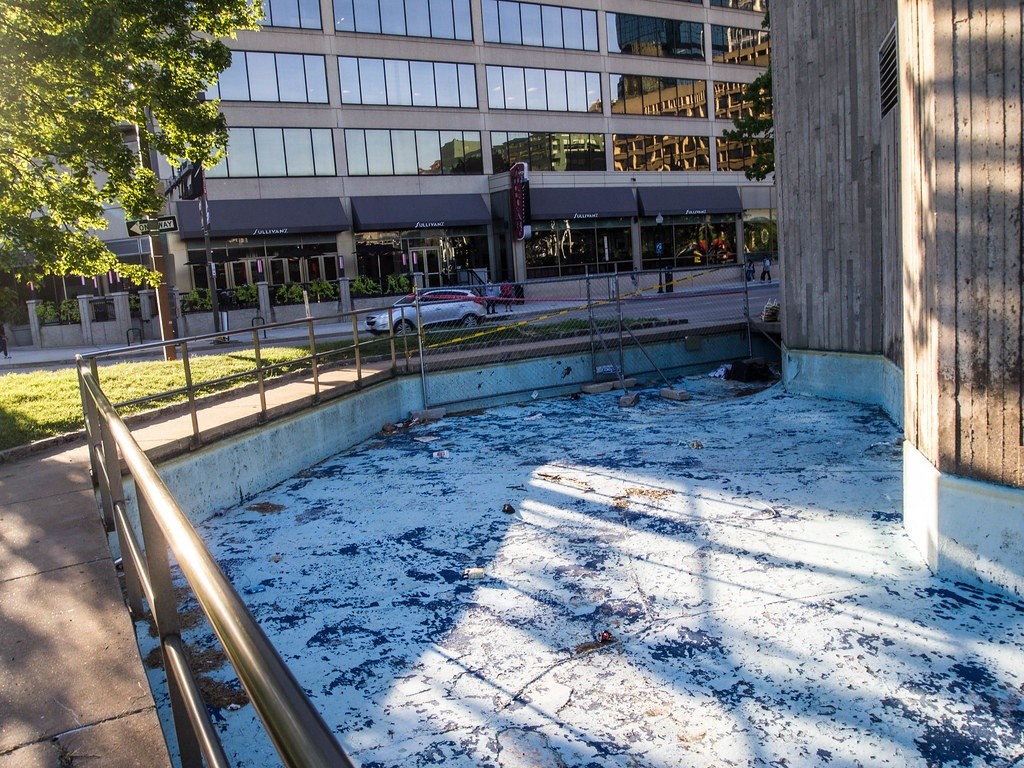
[126,216,179,237]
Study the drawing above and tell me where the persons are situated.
[746,259,755,282]
[485,279,499,314]
[0,323,12,359]
[630,267,639,292]
[500,279,514,312]
[760,256,772,282]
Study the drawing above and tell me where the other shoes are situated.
[4,355,12,359]
[487,311,498,315]
[505,309,514,312]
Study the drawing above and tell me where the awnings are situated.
[351,193,492,233]
[530,187,638,220]
[637,185,742,217]
[175,197,350,240]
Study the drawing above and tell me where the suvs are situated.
[365,290,488,338]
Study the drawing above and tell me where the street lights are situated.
[656,212,664,294]
